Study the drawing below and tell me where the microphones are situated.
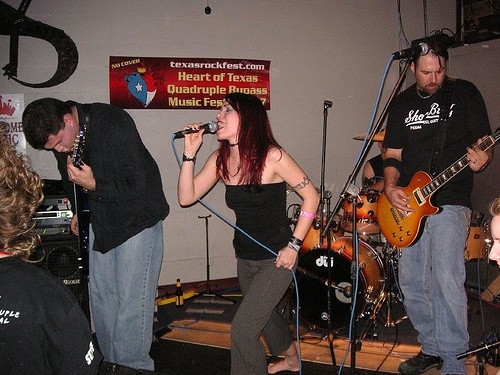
[173,120,218,139]
[344,286,353,298]
[392,43,429,59]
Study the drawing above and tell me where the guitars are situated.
[376,128,500,248]
[72,131,90,283]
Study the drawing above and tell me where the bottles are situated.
[175,278,184,308]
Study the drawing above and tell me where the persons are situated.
[22,98,170,375]
[0,121,103,375]
[362,41,500,375]
[178,93,320,375]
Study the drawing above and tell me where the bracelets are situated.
[288,235,302,252]
[183,153,196,164]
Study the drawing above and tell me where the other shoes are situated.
[398,350,442,374]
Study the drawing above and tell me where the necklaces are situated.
[417,85,432,99]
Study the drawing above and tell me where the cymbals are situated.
[352,131,386,142]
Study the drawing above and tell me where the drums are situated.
[288,236,386,333]
[292,206,346,258]
[342,189,382,235]
[463,219,489,264]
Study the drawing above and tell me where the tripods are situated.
[357,244,407,352]
[186,215,236,303]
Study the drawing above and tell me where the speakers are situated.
[29,238,92,331]
